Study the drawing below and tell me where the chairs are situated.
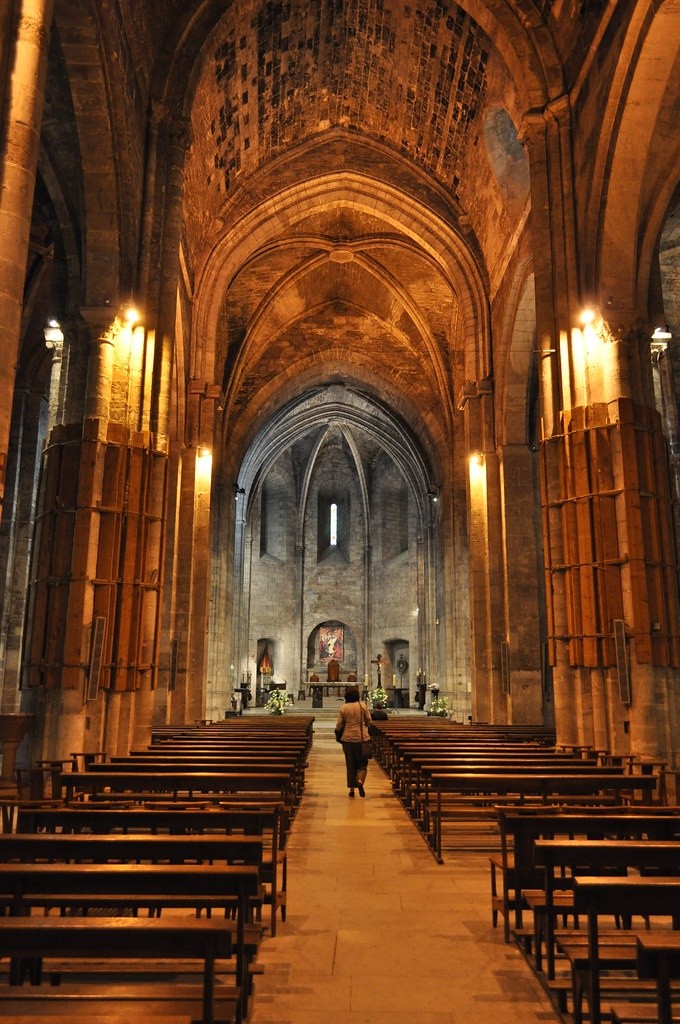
[309,675,319,696]
[194,719,213,726]
[69,800,138,918]
[70,752,110,804]
[619,762,668,806]
[36,759,85,807]
[576,750,610,796]
[560,746,593,759]
[657,771,680,805]
[489,806,560,943]
[326,660,341,696]
[219,802,288,937]
[0,801,65,916]
[597,756,636,807]
[469,722,488,725]
[554,805,628,929]
[346,675,359,688]
[627,806,680,929]
[144,801,212,920]
[12,768,64,863]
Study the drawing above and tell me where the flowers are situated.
[264,690,294,713]
[429,697,454,714]
[429,683,441,690]
[366,688,388,706]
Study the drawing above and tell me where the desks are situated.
[386,688,409,708]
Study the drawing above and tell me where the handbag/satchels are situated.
[361,740,374,759]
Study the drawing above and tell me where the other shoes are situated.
[356,780,365,797]
[349,792,354,797]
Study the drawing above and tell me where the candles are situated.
[365,674,368,686]
[392,674,396,686]
[416,671,419,676]
[419,668,421,673]
[423,670,425,676]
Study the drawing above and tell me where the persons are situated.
[367,702,388,738]
[334,687,371,799]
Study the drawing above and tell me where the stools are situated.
[298,690,305,701]
[287,694,294,704]
[362,691,368,701]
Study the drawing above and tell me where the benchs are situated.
[368,715,680,1024]
[0,715,316,1024]
